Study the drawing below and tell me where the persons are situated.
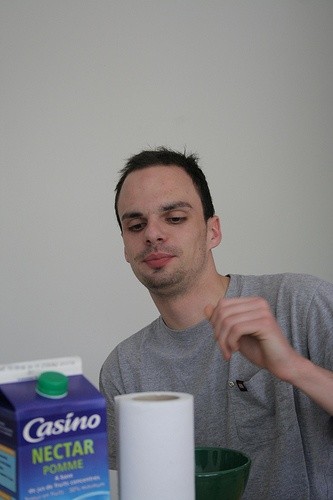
[100,147,333,500]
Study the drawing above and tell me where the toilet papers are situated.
[115,390,195,500]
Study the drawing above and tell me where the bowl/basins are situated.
[195,447,251,500]
